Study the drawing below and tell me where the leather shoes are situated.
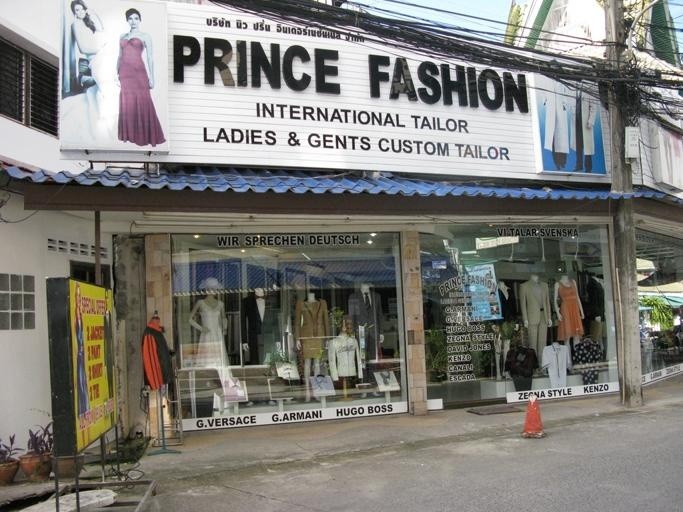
[557,164,591,173]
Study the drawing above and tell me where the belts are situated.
[539,307,544,311]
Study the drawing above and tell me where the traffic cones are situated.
[521,394,547,439]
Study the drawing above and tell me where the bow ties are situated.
[255,295,265,299]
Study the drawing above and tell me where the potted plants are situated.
[0,420,88,487]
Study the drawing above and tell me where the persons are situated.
[71,1,122,148]
[142,316,171,390]
[190,277,384,402]
[543,79,570,169]
[116,9,165,145]
[489,275,605,391]
[567,82,596,173]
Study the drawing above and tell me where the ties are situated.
[364,292,370,309]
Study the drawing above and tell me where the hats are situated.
[198,276,223,290]
